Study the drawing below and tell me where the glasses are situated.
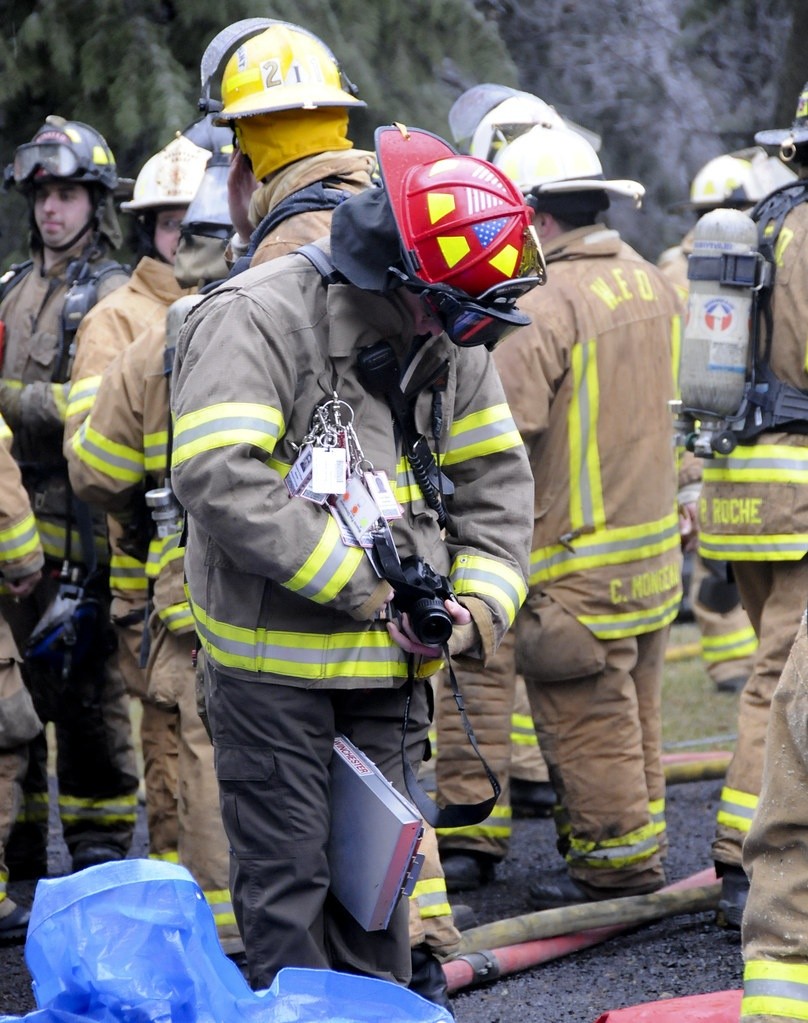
[450,308,532,351]
[14,139,82,185]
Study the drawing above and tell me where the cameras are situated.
[387,556,460,649]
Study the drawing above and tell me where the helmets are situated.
[689,156,756,205]
[180,148,234,231]
[120,130,214,214]
[5,115,119,190]
[469,94,564,168]
[375,122,546,323]
[756,83,808,147]
[493,125,646,205]
[210,24,368,130]
[187,115,234,152]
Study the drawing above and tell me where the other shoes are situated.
[0,903,33,942]
[437,845,498,890]
[716,672,750,694]
[526,869,665,902]
[717,861,751,928]
[401,942,455,1019]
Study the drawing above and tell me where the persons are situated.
[376,478,387,493]
[174,128,545,987]
[0,15,808,1022]
[300,455,312,470]
